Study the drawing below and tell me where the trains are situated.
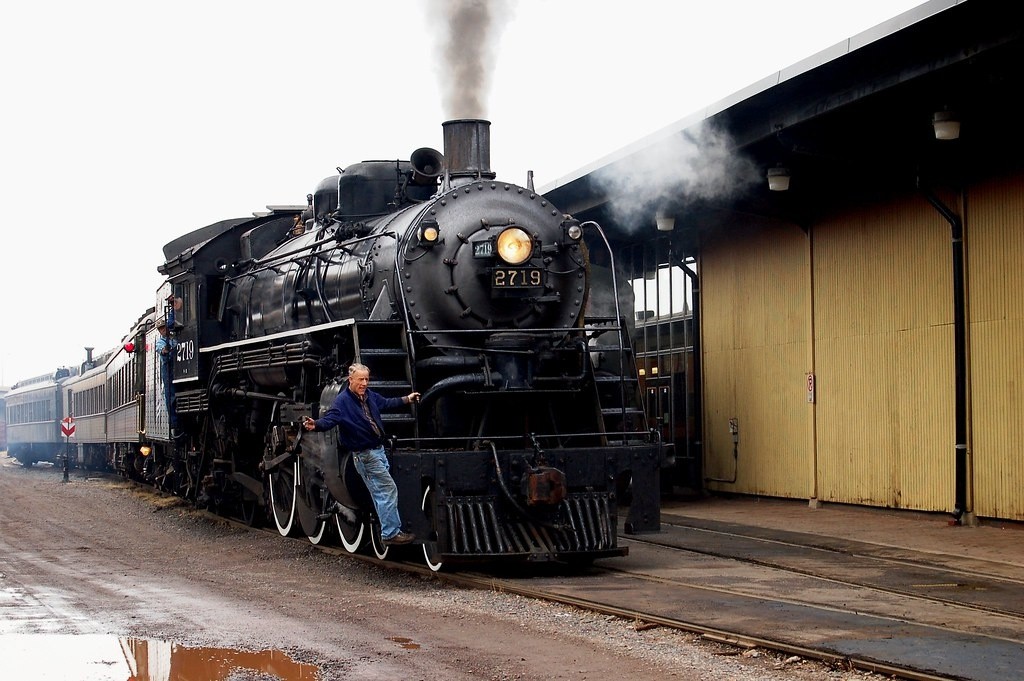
[0,119,678,579]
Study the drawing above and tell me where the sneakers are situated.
[381,531,416,544]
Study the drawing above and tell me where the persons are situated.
[163,290,182,328]
[155,319,178,427]
[303,362,423,544]
